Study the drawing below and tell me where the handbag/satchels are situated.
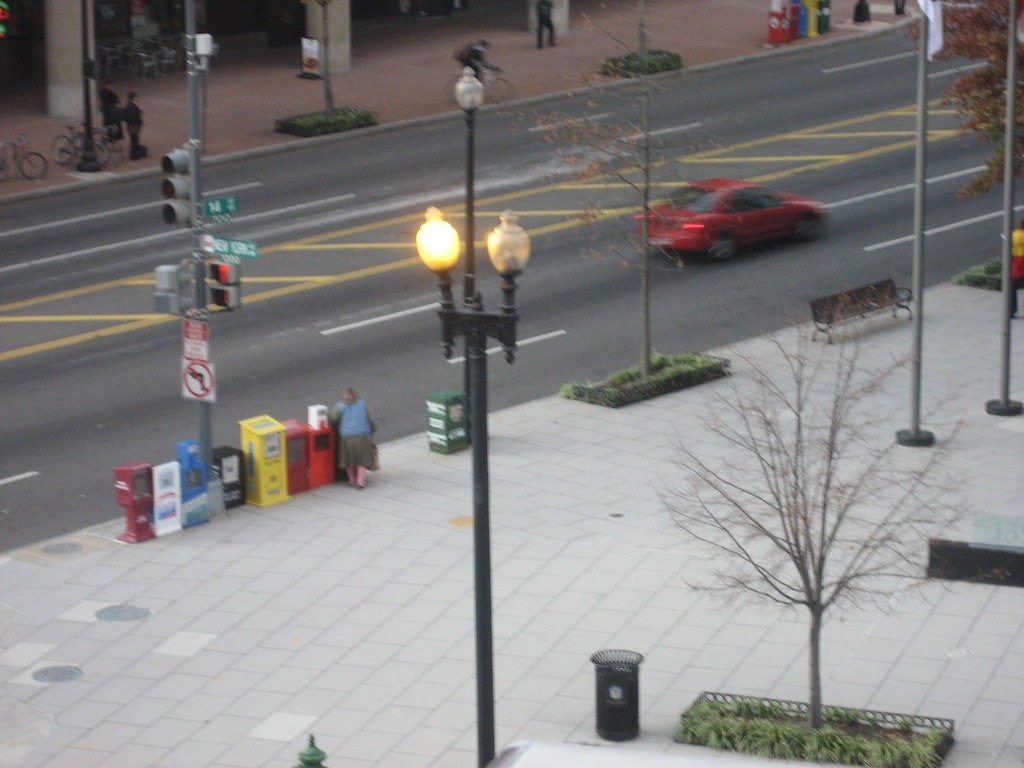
[134,143,147,160]
[366,442,380,472]
[111,102,127,122]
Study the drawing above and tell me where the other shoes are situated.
[548,42,556,46]
[538,44,543,48]
[347,481,356,486]
[357,483,365,488]
[1008,313,1018,320]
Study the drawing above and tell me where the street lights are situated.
[413,66,531,768]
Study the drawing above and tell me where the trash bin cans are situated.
[790,0,807,38]
[768,10,789,43]
[802,0,818,37]
[819,0,831,33]
[591,649,644,742]
[781,4,800,41]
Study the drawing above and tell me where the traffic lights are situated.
[162,148,194,228]
[207,260,241,311]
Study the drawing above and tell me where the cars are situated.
[634,177,828,264]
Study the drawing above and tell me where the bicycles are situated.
[442,68,516,103]
[0,134,50,181]
[51,126,125,170]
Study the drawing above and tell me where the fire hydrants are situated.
[1010,226,1024,282]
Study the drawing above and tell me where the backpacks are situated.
[453,46,469,61]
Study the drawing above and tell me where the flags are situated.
[917,0,943,62]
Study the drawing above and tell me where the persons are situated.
[100,81,124,140]
[330,386,375,488]
[535,0,556,48]
[456,40,502,85]
[124,91,144,160]
[1009,220,1024,318]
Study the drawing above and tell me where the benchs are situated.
[809,278,913,345]
